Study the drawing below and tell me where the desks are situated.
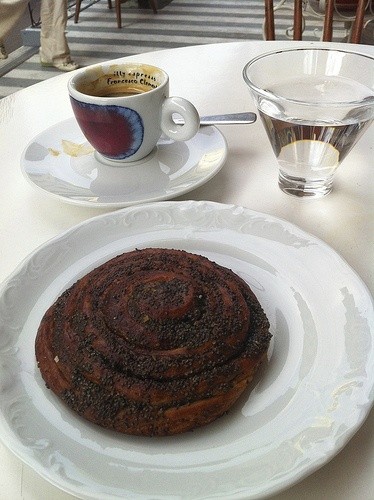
[0,40,374,500]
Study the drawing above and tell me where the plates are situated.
[0,202,374,500]
[20,115,226,206]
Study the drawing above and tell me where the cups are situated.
[68,64,201,168]
[242,47,374,199]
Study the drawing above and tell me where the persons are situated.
[0,0,79,71]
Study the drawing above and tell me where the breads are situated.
[35,248,273,437]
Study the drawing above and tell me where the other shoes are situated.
[0,44,8,59]
[40,56,79,71]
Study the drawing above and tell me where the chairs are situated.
[263,0,374,45]
